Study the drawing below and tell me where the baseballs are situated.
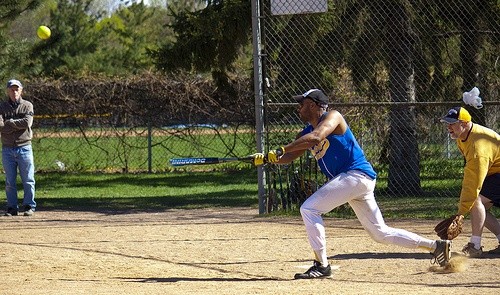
[37,25,51,39]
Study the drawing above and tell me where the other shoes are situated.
[23,208,34,216]
[5,207,19,216]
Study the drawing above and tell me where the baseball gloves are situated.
[435,214,464,240]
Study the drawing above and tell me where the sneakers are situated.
[294,258,332,279]
[488,243,500,255]
[430,239,453,267]
[460,241,484,258]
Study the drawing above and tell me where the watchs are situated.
[276,148,283,157]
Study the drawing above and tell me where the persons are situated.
[0,79,36,217]
[251,89,452,280]
[439,107,500,258]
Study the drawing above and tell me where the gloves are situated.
[249,152,268,167]
[267,146,285,163]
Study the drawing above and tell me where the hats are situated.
[439,106,471,124]
[291,89,329,109]
[6,80,23,90]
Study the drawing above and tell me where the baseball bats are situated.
[169,151,281,166]
